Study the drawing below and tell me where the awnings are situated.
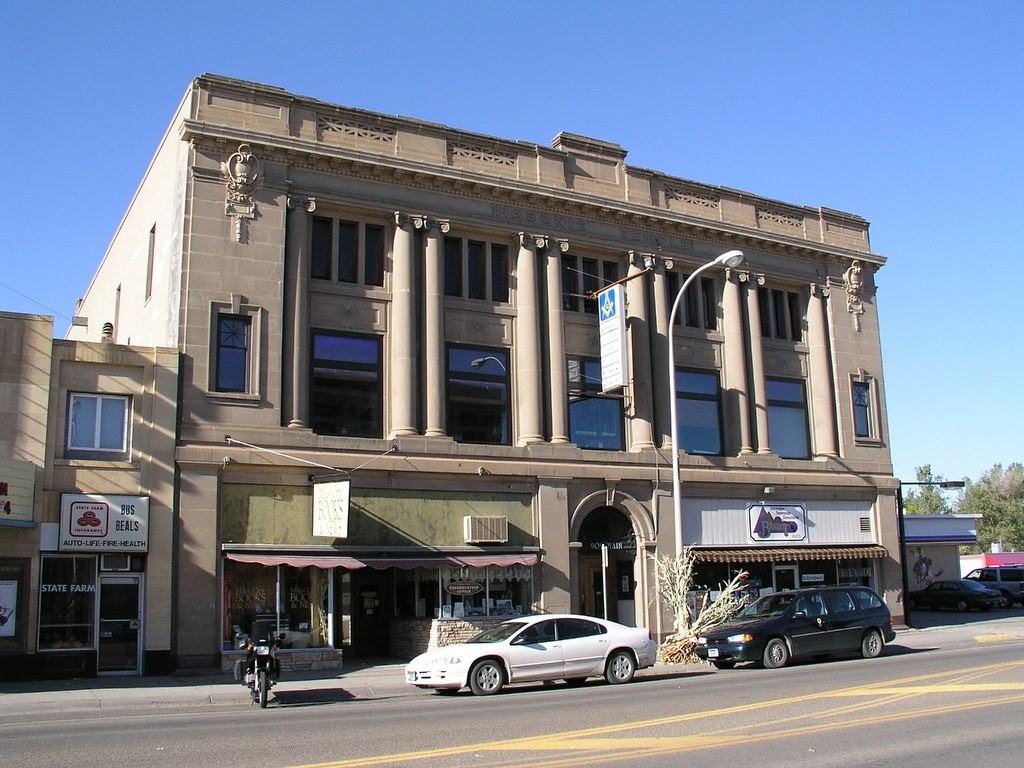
[227,552,538,569]
[686,545,888,561]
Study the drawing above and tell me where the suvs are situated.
[692,584,896,671]
[962,563,1024,609]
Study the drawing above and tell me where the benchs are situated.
[792,612,795,613]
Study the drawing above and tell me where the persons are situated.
[520,627,539,644]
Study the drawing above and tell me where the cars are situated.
[909,578,1002,613]
[403,614,658,697]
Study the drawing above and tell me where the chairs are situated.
[784,599,869,616]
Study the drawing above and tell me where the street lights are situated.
[896,478,966,625]
[668,249,746,634]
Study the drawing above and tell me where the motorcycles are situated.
[233,631,288,709]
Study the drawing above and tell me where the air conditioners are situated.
[463,515,508,544]
